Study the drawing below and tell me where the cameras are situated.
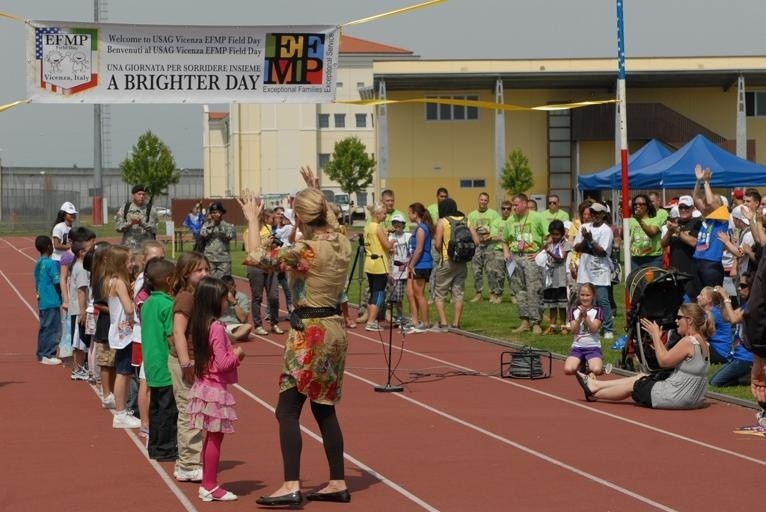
[358,233,365,246]
[270,233,284,246]
[713,284,721,296]
[667,223,690,243]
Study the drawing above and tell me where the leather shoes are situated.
[302,484,352,502]
[255,489,306,509]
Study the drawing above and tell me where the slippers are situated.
[586,370,597,401]
[576,369,595,399]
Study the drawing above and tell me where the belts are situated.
[292,305,344,318]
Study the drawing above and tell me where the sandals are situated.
[199,488,238,503]
[254,328,269,335]
[272,325,285,334]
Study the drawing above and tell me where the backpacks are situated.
[443,216,477,263]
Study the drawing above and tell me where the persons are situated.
[428,188,448,224]
[84,250,94,384]
[404,203,436,333]
[527,198,539,211]
[714,272,762,405]
[573,203,615,340]
[468,193,499,303]
[244,210,285,335]
[139,258,178,459]
[501,195,549,333]
[578,202,595,224]
[492,201,518,303]
[33,235,62,364]
[183,203,207,238]
[61,231,76,314]
[188,277,245,503]
[379,191,404,234]
[133,281,150,433]
[100,244,143,429]
[432,198,480,329]
[70,232,93,381]
[275,208,297,243]
[390,214,411,325]
[607,205,623,315]
[200,203,236,277]
[271,209,284,226]
[651,191,663,212]
[720,195,729,210]
[697,286,756,387]
[577,302,716,410]
[364,202,399,332]
[544,219,572,336]
[234,166,351,508]
[628,194,668,274]
[562,282,605,376]
[91,248,116,406]
[133,240,164,299]
[716,190,762,268]
[113,185,159,245]
[542,194,570,231]
[50,201,78,259]
[691,166,731,288]
[661,195,702,272]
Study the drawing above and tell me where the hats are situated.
[284,209,297,226]
[733,190,745,199]
[391,215,406,224]
[679,195,694,206]
[588,201,608,213]
[132,184,146,193]
[209,200,227,214]
[60,202,79,216]
[731,205,757,228]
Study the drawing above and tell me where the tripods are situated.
[347,247,368,307]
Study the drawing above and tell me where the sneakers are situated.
[429,324,450,334]
[174,466,206,484]
[403,324,416,330]
[40,357,63,368]
[71,369,88,381]
[561,327,569,337]
[603,331,613,340]
[102,397,114,408]
[544,328,558,336]
[103,398,113,407]
[364,322,386,334]
[113,414,143,430]
[413,323,431,333]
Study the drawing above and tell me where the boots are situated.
[512,296,518,305]
[511,318,532,333]
[470,293,483,303]
[356,310,369,322]
[385,310,396,322]
[533,325,542,336]
[494,297,503,303]
[489,292,497,303]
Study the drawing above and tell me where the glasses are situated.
[500,206,513,210]
[633,201,646,207]
[738,282,747,287]
[548,201,557,205]
[678,204,692,210]
[675,313,690,320]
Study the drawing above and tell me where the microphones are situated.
[393,260,408,267]
[371,253,383,259]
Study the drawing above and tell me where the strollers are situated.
[620,266,697,373]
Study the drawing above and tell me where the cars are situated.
[321,188,341,217]
[154,207,172,217]
[335,193,355,224]
